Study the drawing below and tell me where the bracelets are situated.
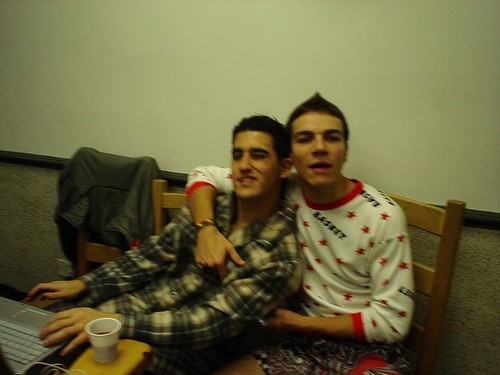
[194,218,217,232]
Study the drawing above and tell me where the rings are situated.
[258,318,266,328]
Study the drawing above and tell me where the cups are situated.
[85,318,123,364]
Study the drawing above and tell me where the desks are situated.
[34,336,155,375]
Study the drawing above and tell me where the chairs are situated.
[23,146,162,309]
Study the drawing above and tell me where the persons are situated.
[22,115,304,375]
[185,93,415,375]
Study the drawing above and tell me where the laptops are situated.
[0,296,75,375]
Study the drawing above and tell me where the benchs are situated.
[153,178,465,375]
